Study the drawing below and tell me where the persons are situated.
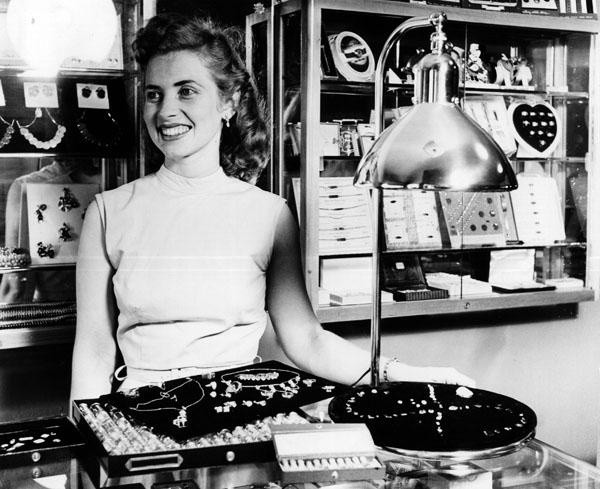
[1,155,122,307]
[69,15,477,431]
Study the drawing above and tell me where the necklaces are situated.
[220,367,301,397]
[0,115,15,152]
[75,109,124,151]
[129,377,206,429]
[16,107,68,151]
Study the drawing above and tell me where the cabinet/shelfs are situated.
[68,439,600,489]
[0,0,143,489]
[244,0,600,328]
